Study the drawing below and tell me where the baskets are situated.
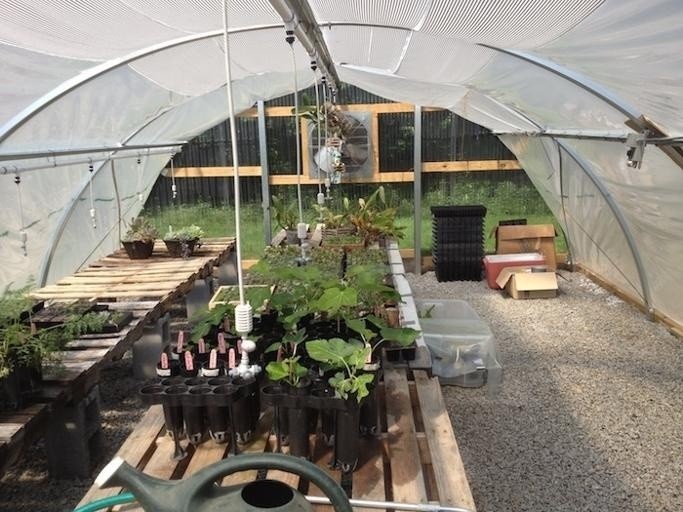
[430,204,487,282]
[122,237,199,259]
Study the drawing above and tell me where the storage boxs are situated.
[482,224,569,300]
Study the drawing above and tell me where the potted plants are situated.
[292,93,353,174]
[120,217,206,259]
[0,282,121,403]
[188,185,423,471]
